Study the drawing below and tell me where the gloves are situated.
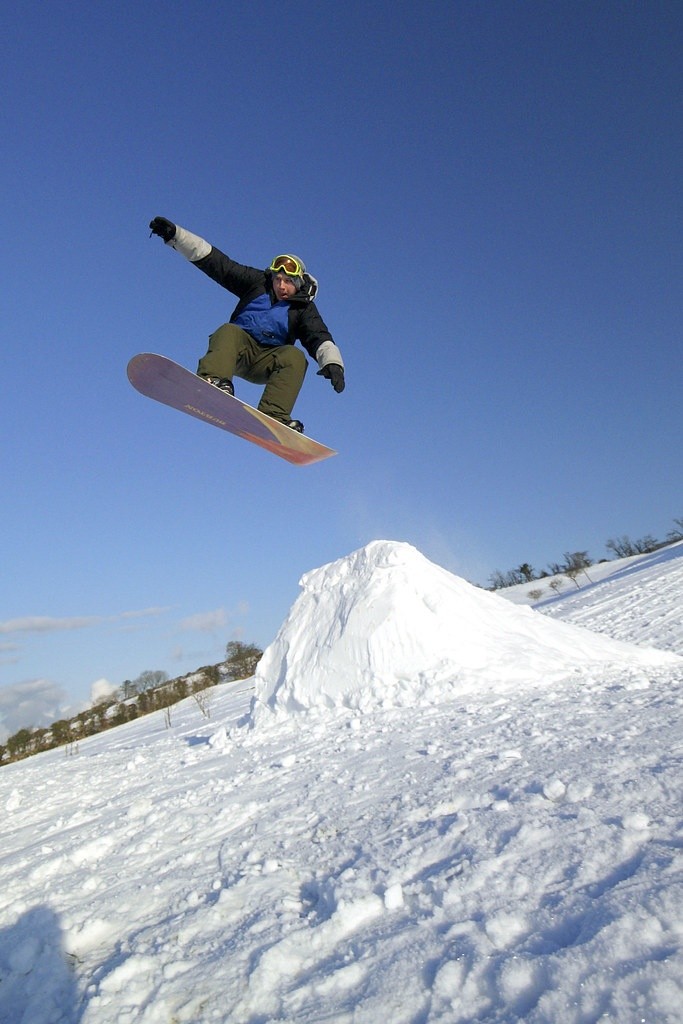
[149,217,176,243]
[316,363,345,393]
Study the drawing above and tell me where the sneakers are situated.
[284,419,306,435]
[214,379,235,396]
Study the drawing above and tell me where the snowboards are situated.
[127,352,336,469]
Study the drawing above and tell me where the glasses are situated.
[270,255,304,277]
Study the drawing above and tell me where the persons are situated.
[148,216,346,432]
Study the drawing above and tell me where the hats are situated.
[282,254,307,272]
